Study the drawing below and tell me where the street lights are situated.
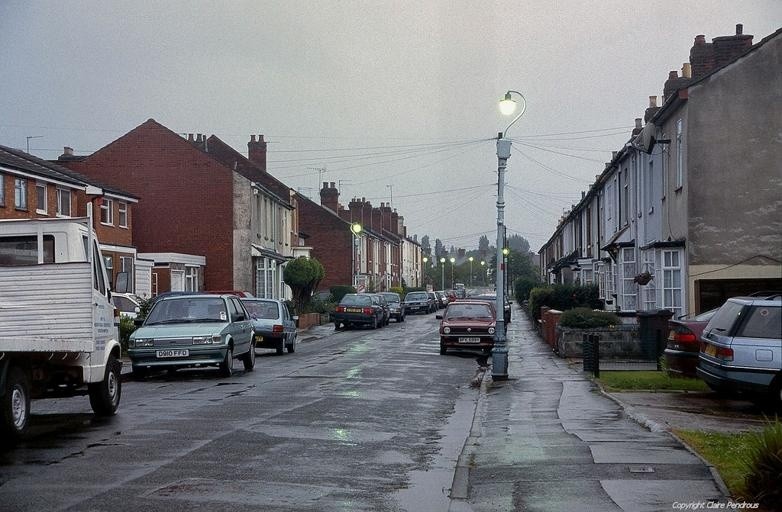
[419,257,487,289]
[349,222,361,294]
[483,87,533,380]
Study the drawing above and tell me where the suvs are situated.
[695,296,782,404]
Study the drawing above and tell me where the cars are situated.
[111,286,297,379]
[335,281,513,356]
[662,292,753,383]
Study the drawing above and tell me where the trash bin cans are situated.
[582,331,601,378]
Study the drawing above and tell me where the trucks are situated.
[0,214,130,436]
